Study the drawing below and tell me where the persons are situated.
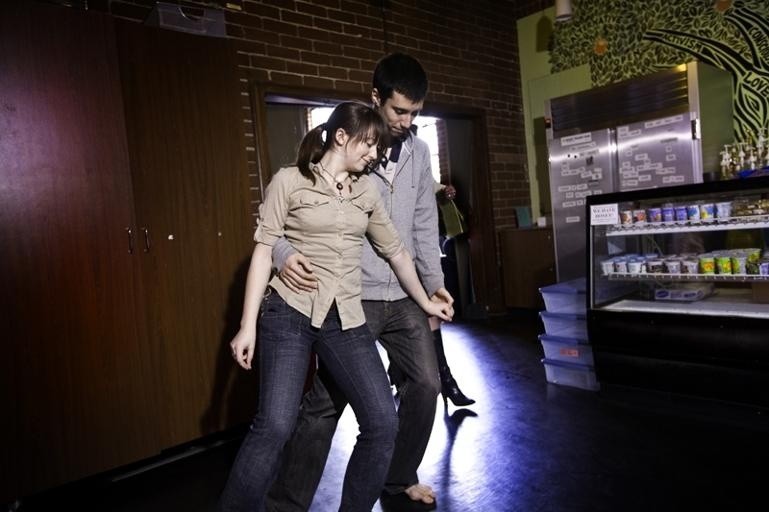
[385,184,475,408]
[212,101,455,510]
[270,50,458,510]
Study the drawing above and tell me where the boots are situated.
[432,327,476,405]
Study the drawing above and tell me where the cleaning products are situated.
[717,126,769,181]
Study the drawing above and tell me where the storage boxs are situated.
[538,276,601,391]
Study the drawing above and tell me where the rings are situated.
[448,193,451,198]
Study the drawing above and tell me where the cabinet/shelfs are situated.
[585,176,769,323]
[0,1,258,499]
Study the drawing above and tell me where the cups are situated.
[614,202,731,225]
[602,252,659,273]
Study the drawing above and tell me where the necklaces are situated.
[318,161,351,190]
